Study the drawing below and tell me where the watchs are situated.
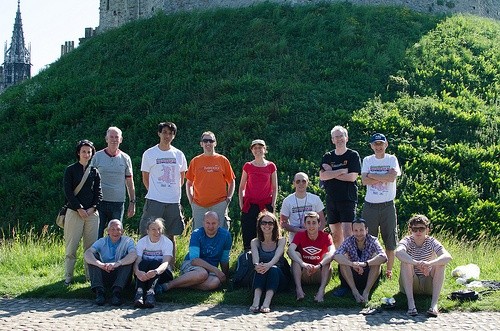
[130,199,136,203]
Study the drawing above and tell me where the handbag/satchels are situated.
[56,205,68,228]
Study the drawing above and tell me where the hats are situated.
[370,133,386,144]
[251,139,266,148]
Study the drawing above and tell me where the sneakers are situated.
[134,291,144,307]
[146,291,155,307]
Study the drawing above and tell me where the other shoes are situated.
[154,283,167,295]
[96,290,105,305]
[386,271,392,277]
[112,290,122,305]
[65,279,72,285]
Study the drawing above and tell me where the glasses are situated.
[201,139,216,143]
[260,221,274,226]
[411,226,427,232]
[79,140,93,146]
[332,135,345,140]
[294,179,309,184]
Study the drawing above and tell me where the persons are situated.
[91,126,136,240]
[332,219,389,306]
[156,210,232,302]
[131,217,174,309]
[239,139,278,252]
[280,172,326,245]
[84,219,138,306]
[247,212,288,313]
[185,131,236,228]
[139,121,188,270]
[394,215,452,317]
[286,211,336,302]
[318,125,361,250]
[62,140,104,288]
[361,133,401,280]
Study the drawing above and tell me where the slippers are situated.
[249,305,259,312]
[407,308,418,316]
[427,307,438,316]
[260,305,270,313]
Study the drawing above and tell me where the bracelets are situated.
[319,262,322,269]
[364,260,368,265]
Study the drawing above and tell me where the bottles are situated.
[381,297,396,305]
